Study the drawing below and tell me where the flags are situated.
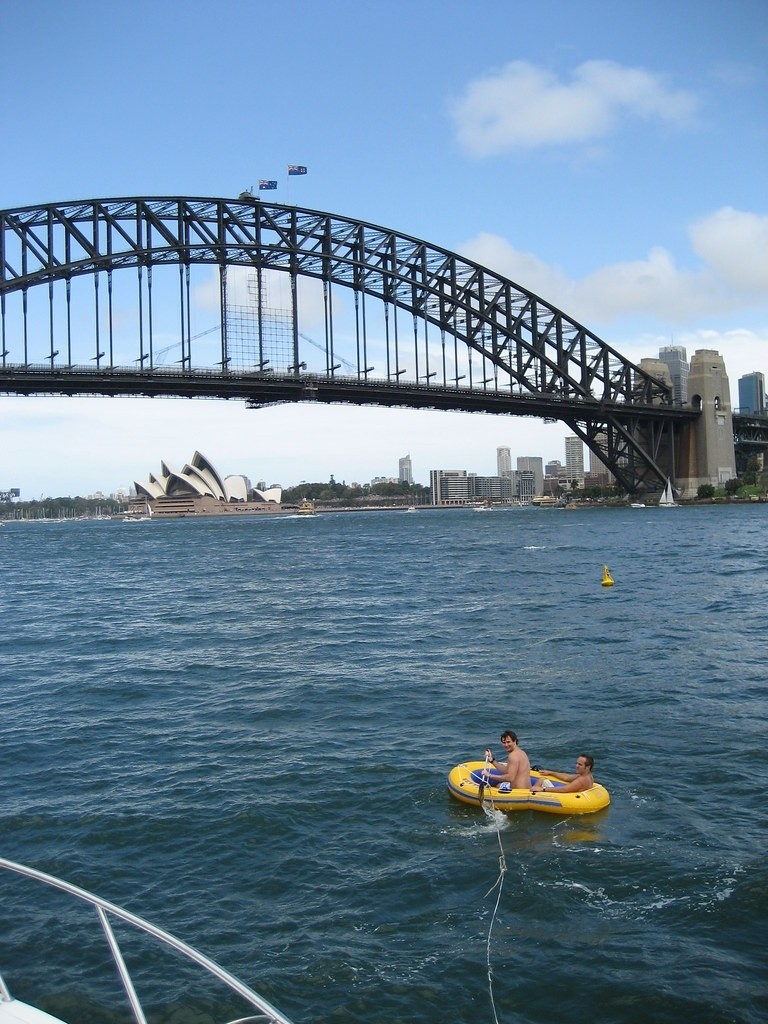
[288,165,307,176]
[259,180,277,189]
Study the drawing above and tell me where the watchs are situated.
[543,787,546,791]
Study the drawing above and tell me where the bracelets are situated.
[488,774,491,778]
[490,758,495,763]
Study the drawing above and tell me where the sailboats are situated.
[658,475,680,508]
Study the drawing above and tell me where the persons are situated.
[530,754,594,794]
[481,730,532,789]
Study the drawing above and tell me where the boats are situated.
[448,760,612,816]
[298,501,317,516]
[631,503,646,508]
[602,565,614,585]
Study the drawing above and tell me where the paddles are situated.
[478,749,489,807]
